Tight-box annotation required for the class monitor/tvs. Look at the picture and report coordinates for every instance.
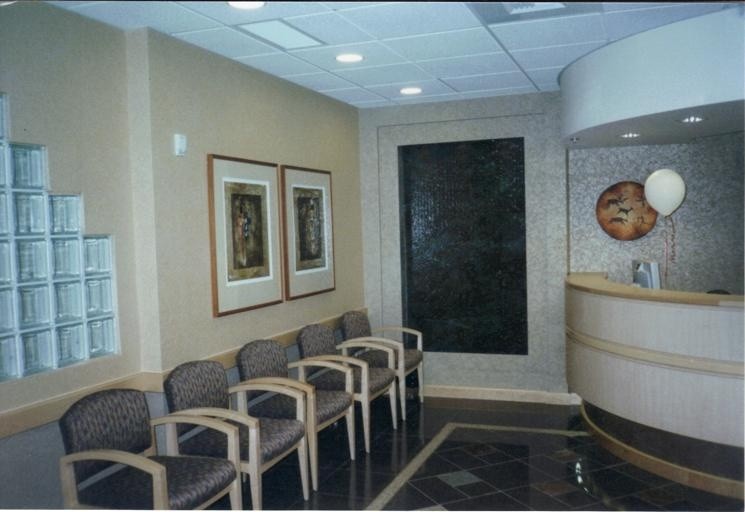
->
[632,259,661,290]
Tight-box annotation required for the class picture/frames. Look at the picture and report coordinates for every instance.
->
[208,154,287,318]
[281,164,336,301]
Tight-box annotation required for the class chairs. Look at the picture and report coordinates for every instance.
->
[164,360,310,510]
[59,389,245,510]
[297,324,399,453]
[234,340,357,492]
[340,311,424,421]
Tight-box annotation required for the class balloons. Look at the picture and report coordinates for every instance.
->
[643,169,686,218]
